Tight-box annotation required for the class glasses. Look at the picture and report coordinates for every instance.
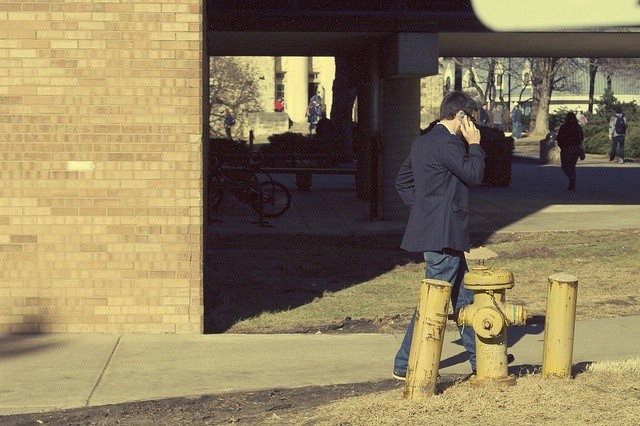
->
[463,109,476,124]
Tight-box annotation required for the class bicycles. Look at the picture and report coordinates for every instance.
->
[209,150,292,218]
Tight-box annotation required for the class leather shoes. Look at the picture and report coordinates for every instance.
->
[393,368,441,381]
[471,353,515,376]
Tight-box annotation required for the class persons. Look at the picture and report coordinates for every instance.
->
[556,112,584,190]
[480,104,489,127]
[223,109,235,139]
[392,91,514,381]
[273,97,284,112]
[510,101,522,138]
[304,91,326,134]
[578,111,588,125]
[574,110,581,120]
[609,106,626,164]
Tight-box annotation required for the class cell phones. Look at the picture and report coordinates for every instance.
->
[458,112,471,128]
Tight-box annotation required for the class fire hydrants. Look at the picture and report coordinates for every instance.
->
[448,258,533,386]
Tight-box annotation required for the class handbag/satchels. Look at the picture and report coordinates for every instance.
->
[579,147,585,160]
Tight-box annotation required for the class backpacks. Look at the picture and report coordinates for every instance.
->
[614,113,626,133]
